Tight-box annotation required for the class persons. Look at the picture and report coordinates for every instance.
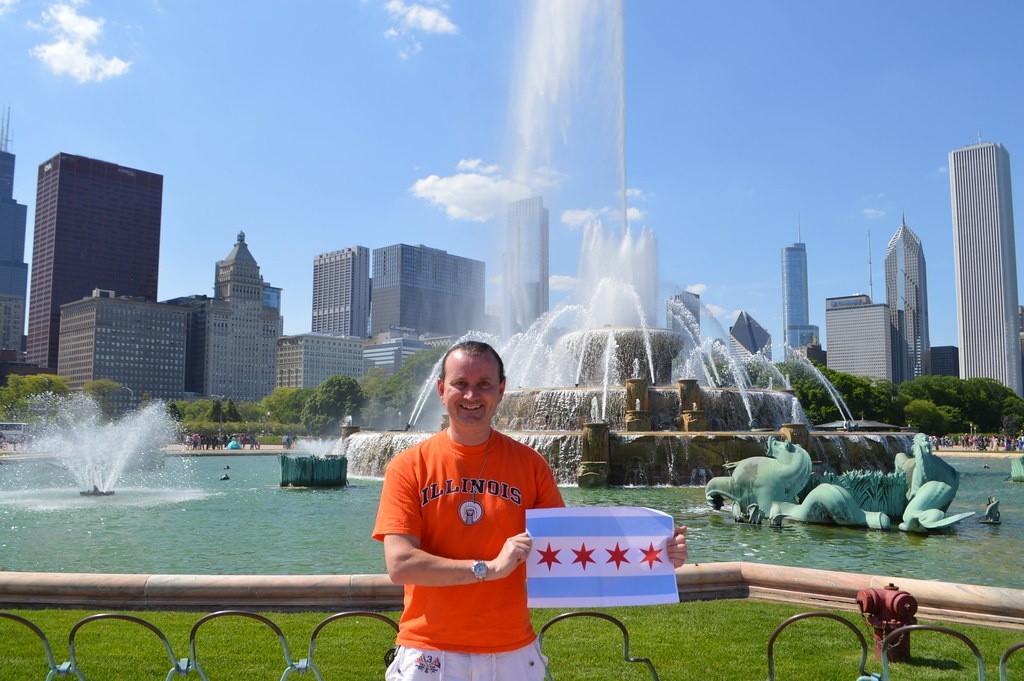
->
[176,431,332,450]
[373,341,688,681]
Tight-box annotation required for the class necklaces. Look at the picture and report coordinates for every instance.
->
[447,426,493,506]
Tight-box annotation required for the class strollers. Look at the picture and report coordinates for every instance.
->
[977,439,987,451]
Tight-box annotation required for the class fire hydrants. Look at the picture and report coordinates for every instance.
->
[855,582,919,664]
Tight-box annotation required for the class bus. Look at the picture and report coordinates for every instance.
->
[0,423,30,446]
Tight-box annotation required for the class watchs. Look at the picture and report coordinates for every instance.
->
[472,559,488,582]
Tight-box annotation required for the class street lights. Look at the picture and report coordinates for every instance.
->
[123,387,133,411]
[969,422,973,436]
[267,411,271,436]
[211,395,224,437]
[974,427,977,437]
